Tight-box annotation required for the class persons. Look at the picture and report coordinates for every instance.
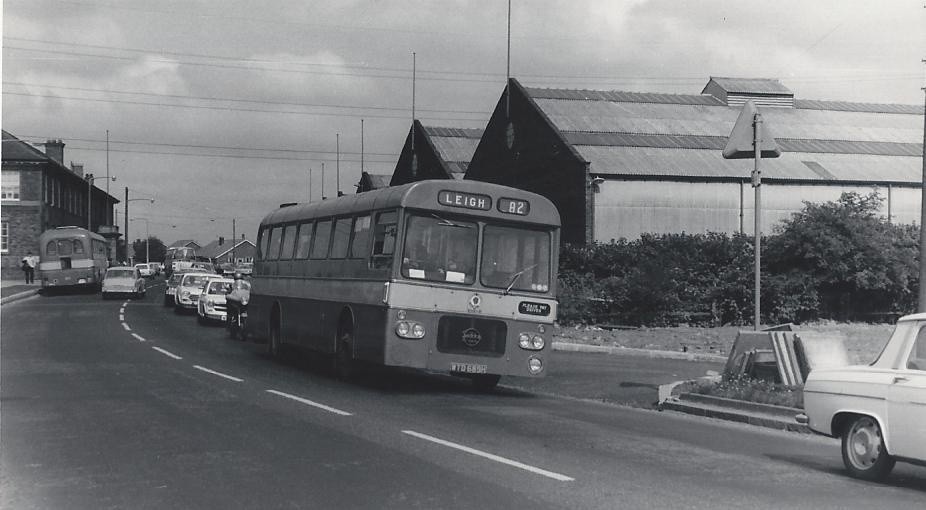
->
[224,273,245,329]
[19,250,37,284]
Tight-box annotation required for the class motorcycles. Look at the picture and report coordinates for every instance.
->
[226,305,249,341]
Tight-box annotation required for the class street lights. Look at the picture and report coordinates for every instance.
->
[86,175,116,232]
[208,218,236,259]
[114,198,155,230]
[128,218,150,268]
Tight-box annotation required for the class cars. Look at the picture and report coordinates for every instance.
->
[793,312,926,481]
[101,254,254,325]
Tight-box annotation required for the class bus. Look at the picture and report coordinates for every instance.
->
[38,225,110,290]
[240,179,563,389]
